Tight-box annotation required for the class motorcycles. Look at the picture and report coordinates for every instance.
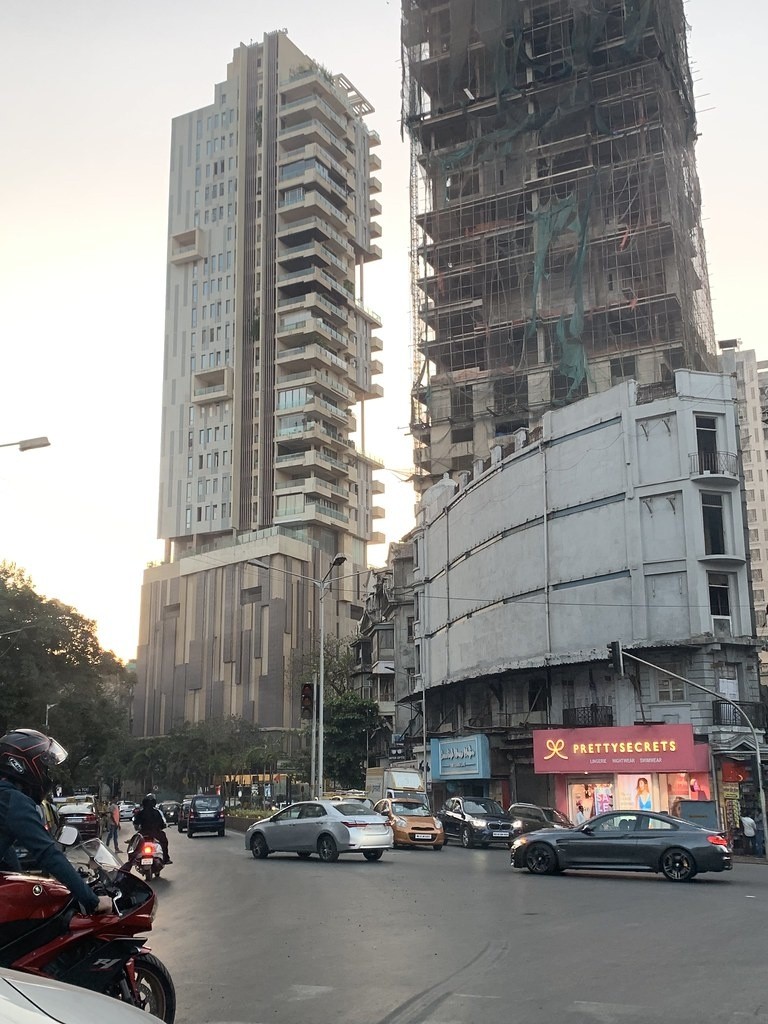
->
[0,827,178,1024]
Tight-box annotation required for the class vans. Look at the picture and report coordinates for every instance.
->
[187,793,226,838]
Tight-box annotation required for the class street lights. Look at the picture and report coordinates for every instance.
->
[384,666,427,790]
[246,551,395,803]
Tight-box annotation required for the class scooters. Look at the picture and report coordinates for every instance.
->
[128,817,171,881]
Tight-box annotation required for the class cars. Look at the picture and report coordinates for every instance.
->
[507,802,576,833]
[158,802,181,825]
[58,802,105,845]
[177,795,209,834]
[330,795,376,810]
[244,801,394,863]
[15,800,56,877]
[372,798,443,850]
[117,800,136,822]
[509,809,736,882]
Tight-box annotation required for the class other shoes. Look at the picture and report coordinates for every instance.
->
[164,860,172,864]
[115,849,123,853]
[755,855,763,859]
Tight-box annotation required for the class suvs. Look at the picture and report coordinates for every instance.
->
[435,795,522,850]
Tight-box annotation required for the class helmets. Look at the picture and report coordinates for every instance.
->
[142,794,156,807]
[0,728,69,806]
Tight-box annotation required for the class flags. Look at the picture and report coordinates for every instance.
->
[274,774,280,781]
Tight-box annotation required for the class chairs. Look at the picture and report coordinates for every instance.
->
[619,819,636,830]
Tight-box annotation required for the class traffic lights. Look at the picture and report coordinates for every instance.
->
[299,681,313,720]
[606,642,625,676]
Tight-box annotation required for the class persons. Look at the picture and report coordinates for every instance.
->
[635,778,653,829]
[0,728,112,916]
[740,807,768,858]
[619,819,630,829]
[133,792,173,864]
[106,795,123,853]
[577,806,585,824]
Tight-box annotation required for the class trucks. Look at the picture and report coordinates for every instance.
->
[366,767,431,813]
